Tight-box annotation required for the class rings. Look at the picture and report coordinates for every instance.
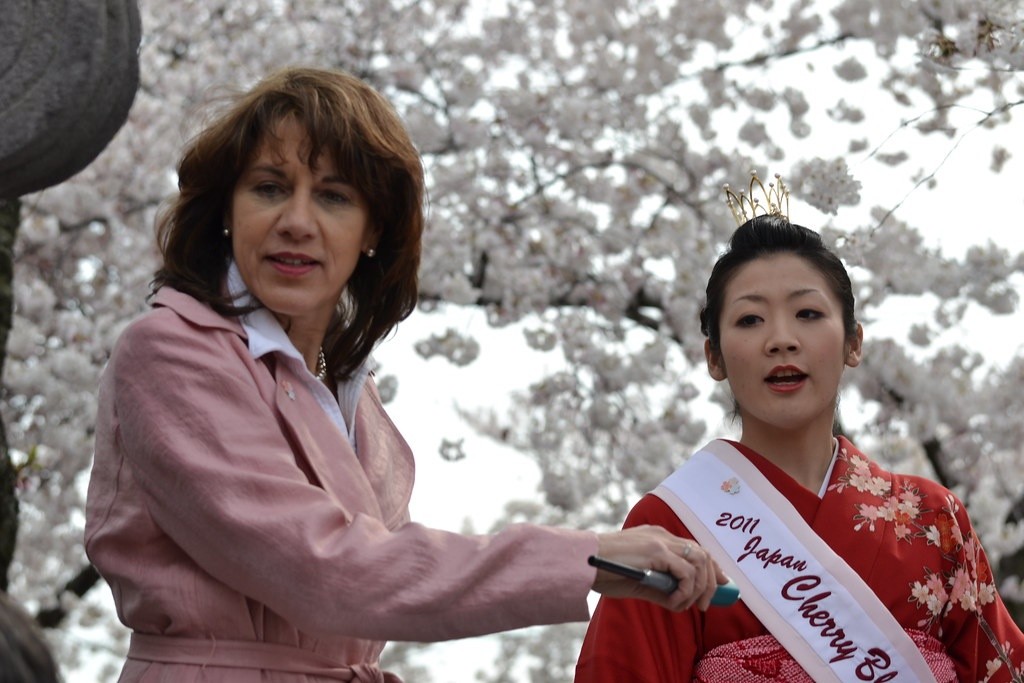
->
[683,544,692,559]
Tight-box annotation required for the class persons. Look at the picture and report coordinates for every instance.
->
[85,64,728,683]
[574,170,1024,683]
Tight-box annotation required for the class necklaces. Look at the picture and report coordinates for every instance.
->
[313,345,328,383]
